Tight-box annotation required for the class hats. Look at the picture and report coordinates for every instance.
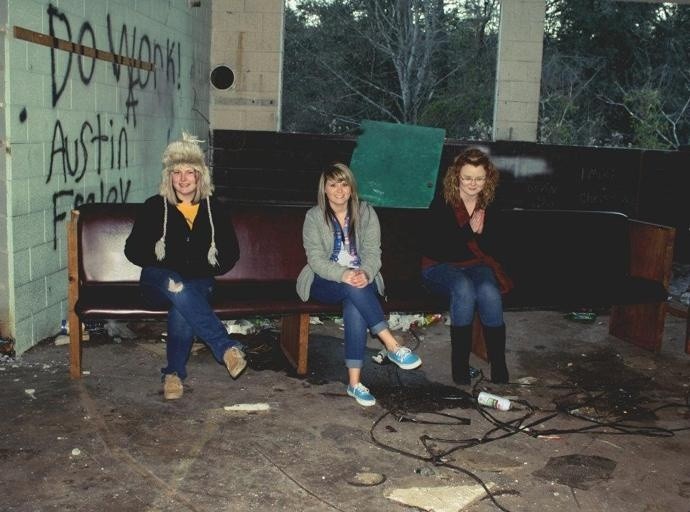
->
[160,129,214,201]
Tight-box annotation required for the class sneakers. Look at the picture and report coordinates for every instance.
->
[347,383,376,407]
[163,374,184,400]
[387,346,422,370]
[222,346,248,379]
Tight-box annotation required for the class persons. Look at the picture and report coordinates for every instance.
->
[418,149,513,385]
[124,140,248,401]
[295,162,423,407]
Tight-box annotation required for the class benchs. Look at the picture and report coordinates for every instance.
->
[67,203,677,378]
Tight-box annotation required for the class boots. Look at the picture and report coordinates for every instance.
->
[483,323,509,385]
[451,322,472,385]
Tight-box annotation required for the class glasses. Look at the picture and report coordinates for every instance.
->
[458,175,486,185]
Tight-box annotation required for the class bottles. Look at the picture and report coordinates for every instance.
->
[563,311,597,321]
[476,391,514,412]
